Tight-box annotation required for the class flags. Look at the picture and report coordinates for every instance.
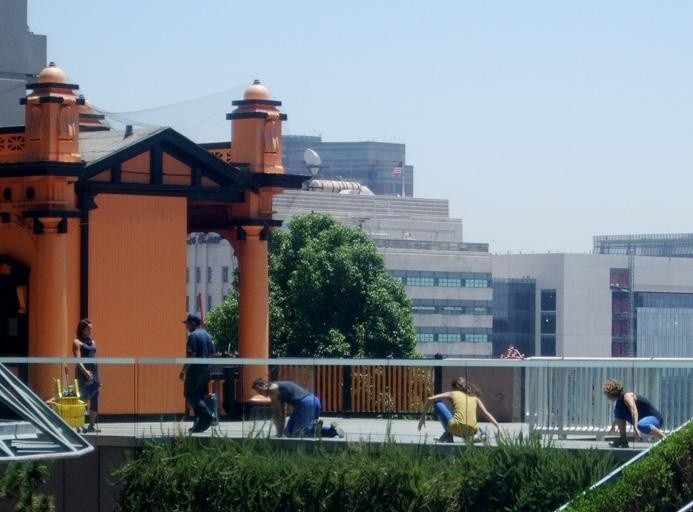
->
[392,158,402,176]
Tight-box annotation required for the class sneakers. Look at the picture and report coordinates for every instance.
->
[434,435,453,443]
[88,425,102,433]
[331,421,345,437]
[608,438,628,448]
[189,412,218,433]
[314,419,323,438]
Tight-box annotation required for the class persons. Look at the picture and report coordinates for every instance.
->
[601,377,665,449]
[178,312,218,433]
[71,318,102,433]
[249,375,346,439]
[416,375,504,444]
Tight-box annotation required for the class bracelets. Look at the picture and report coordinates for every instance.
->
[421,416,425,420]
[180,370,184,373]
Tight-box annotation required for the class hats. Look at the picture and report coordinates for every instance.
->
[182,314,202,326]
